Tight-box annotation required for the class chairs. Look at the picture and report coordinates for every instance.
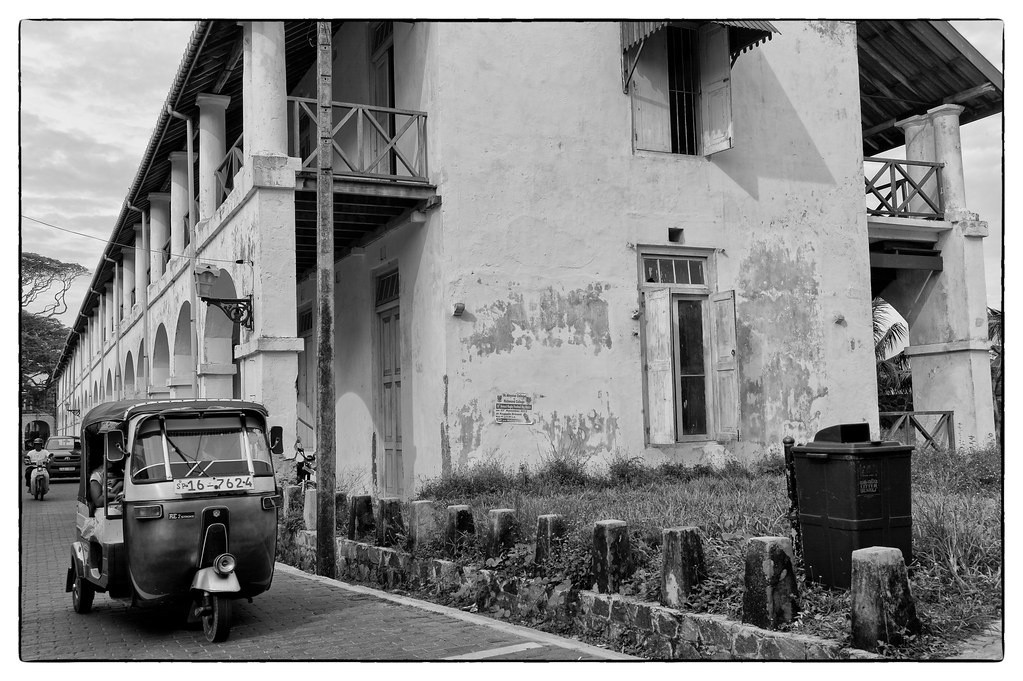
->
[49,441,60,449]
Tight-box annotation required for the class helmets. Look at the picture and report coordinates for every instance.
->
[33,438,44,447]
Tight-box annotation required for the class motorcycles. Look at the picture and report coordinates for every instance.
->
[24,452,54,501]
[66,399,285,644]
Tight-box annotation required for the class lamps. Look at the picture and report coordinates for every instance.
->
[452,302,465,316]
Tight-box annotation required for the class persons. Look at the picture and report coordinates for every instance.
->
[86,459,125,516]
[24,438,52,493]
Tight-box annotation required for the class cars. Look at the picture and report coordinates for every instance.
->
[45,435,82,478]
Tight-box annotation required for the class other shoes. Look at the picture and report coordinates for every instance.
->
[27,488,31,493]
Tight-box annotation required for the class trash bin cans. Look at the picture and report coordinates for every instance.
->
[790,423,917,593]
